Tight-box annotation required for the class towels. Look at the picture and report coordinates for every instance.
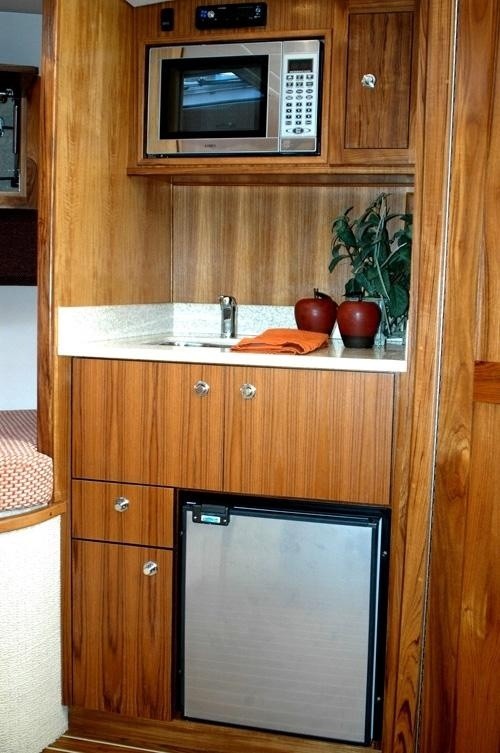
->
[231,328,330,354]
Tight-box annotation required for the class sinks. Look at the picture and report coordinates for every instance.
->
[141,337,242,349]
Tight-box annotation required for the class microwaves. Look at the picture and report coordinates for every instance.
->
[145,38,320,157]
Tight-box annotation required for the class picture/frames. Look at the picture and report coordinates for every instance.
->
[0,78,28,197]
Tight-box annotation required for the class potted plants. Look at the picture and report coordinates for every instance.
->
[329,190,407,349]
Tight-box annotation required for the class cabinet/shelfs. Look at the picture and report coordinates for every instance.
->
[70,477,176,723]
[38,0,498,374]
[70,357,395,509]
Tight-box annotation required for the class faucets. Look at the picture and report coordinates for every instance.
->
[217,295,238,338]
